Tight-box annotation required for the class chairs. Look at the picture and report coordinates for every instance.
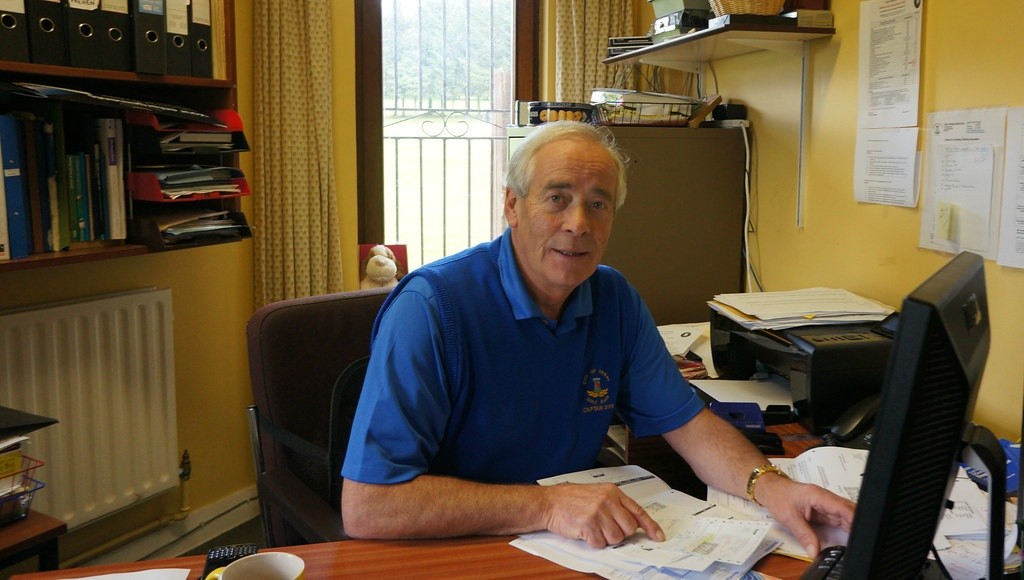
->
[247,290,395,548]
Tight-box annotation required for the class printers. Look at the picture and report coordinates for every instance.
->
[710,308,900,437]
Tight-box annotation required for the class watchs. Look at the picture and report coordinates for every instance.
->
[743,464,792,509]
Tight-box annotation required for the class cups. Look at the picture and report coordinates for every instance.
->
[206,550,306,580]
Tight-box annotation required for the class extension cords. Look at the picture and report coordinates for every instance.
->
[698,119,750,128]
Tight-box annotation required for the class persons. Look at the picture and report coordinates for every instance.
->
[338,119,861,563]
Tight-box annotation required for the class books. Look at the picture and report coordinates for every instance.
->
[0,113,136,261]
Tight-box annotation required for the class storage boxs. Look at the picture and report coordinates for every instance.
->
[646,0,785,46]
[0,475,46,529]
[588,101,693,127]
[0,455,45,499]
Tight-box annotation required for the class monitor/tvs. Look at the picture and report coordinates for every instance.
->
[841,251,990,580]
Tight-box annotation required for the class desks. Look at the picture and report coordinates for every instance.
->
[11,335,1024,580]
[0,503,68,580]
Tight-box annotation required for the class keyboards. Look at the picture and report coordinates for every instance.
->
[799,545,848,580]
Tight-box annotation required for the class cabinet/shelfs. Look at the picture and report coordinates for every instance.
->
[1,0,251,270]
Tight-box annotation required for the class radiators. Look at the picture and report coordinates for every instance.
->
[1,286,182,535]
